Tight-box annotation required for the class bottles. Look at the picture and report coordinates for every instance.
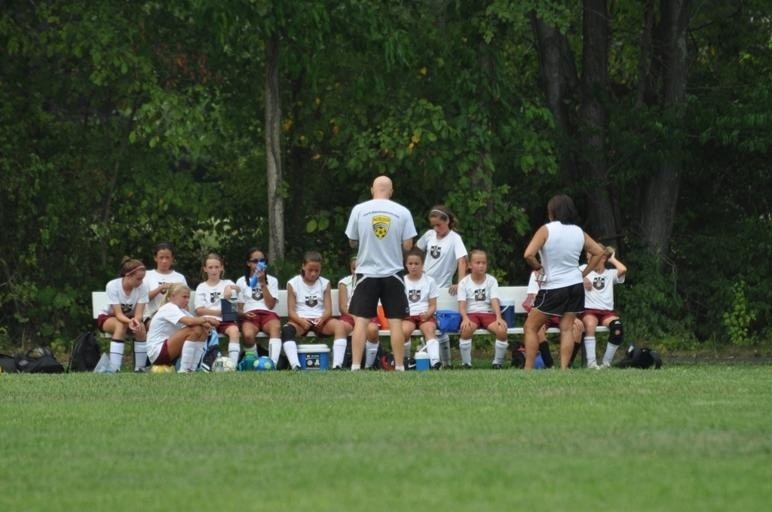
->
[250,260,267,288]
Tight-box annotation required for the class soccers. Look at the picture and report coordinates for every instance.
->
[252,356,276,371]
[211,355,236,374]
[151,364,177,375]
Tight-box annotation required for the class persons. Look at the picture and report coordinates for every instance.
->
[338,253,381,369]
[236,247,282,370]
[578,241,627,369]
[195,253,241,372]
[282,251,348,371]
[413,206,469,370]
[523,196,604,369]
[522,268,585,369]
[344,177,419,372]
[141,243,194,372]
[144,282,219,373]
[96,254,149,373]
[456,249,508,369]
[399,246,444,371]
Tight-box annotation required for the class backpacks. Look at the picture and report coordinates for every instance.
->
[617,345,662,369]
[67,330,101,373]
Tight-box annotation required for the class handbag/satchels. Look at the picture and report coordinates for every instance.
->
[15,341,65,373]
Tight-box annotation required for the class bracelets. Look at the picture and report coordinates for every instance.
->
[535,265,541,272]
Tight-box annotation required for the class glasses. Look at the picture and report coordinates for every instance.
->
[248,258,266,263]
